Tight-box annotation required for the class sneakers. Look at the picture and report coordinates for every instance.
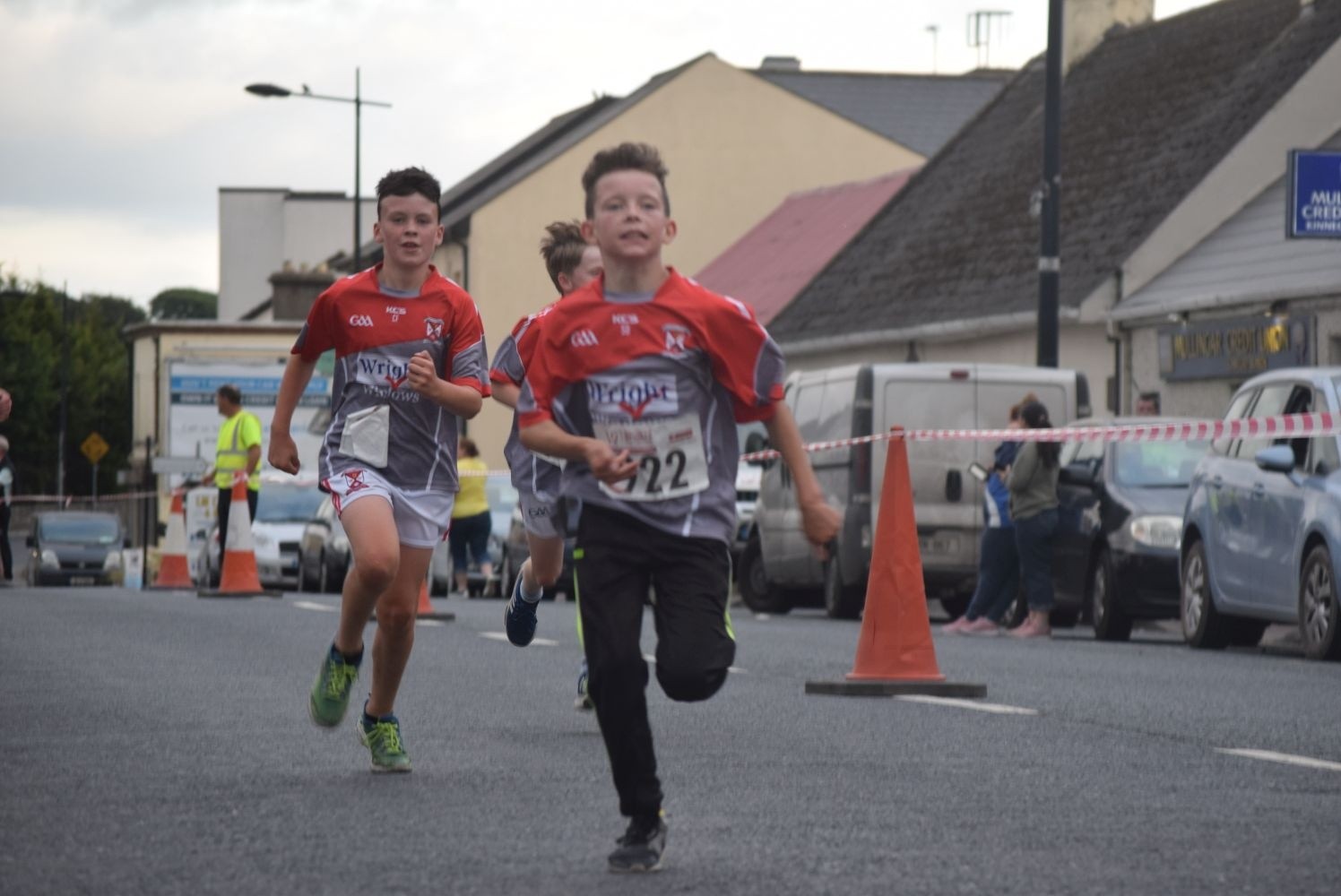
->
[309,636,364,726]
[357,694,412,773]
[607,812,669,872]
[573,666,594,709]
[504,566,540,647]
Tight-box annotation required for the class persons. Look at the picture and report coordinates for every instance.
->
[0,435,12,586]
[200,384,263,588]
[442,437,498,601]
[514,142,842,873]
[940,392,1063,638]
[269,166,492,773]
[489,218,604,711]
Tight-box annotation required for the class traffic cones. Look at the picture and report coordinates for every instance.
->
[198,469,282,600]
[803,425,990,699]
[149,496,198,591]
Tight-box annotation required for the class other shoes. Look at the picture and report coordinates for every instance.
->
[448,590,469,599]
[483,578,496,597]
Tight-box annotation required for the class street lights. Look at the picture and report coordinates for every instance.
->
[243,64,394,276]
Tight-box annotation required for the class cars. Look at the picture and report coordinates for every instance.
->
[1175,364,1341,660]
[23,510,130,587]
[1057,414,1220,642]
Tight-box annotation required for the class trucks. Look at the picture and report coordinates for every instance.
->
[245,360,1084,619]
